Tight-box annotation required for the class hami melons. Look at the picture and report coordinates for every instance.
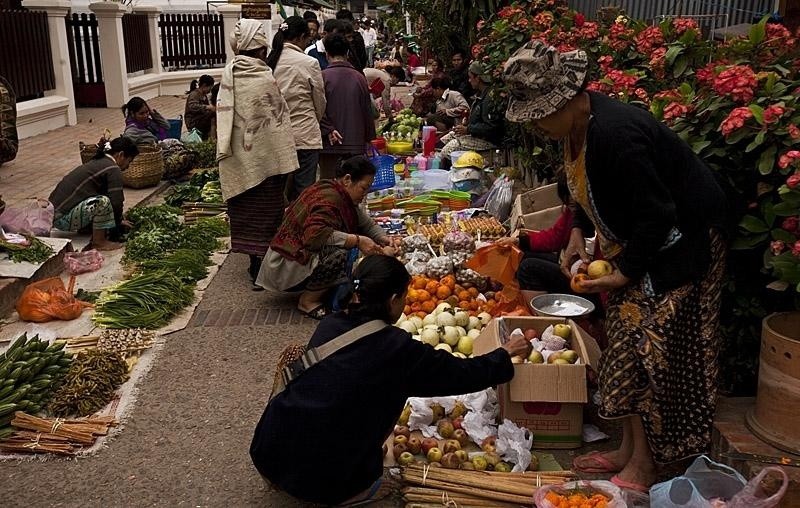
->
[392,302,491,359]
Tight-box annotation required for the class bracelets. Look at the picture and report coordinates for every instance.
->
[355,234,360,247]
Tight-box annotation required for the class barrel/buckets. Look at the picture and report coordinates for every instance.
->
[168,118,182,141]
[371,139,386,155]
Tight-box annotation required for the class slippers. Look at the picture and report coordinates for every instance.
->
[573,450,622,473]
[610,472,661,493]
[298,303,333,320]
[342,478,385,506]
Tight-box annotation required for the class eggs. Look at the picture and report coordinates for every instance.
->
[412,216,507,251]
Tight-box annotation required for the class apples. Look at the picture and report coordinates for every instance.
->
[508,324,577,364]
[587,259,612,280]
[393,399,540,472]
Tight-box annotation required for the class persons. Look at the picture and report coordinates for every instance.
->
[0,74,19,218]
[185,75,222,141]
[121,97,181,145]
[410,52,504,146]
[47,136,139,250]
[502,39,727,504]
[267,9,379,189]
[514,168,609,323]
[254,155,399,319]
[215,19,300,290]
[249,254,533,508]
[363,44,421,121]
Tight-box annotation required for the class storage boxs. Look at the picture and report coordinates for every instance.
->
[471,315,605,449]
[507,178,566,234]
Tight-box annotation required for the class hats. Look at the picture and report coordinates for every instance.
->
[467,60,492,82]
[501,38,589,123]
[451,150,484,195]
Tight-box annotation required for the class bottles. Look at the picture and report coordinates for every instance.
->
[383,131,413,143]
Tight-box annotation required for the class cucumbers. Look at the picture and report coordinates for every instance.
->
[0,332,76,439]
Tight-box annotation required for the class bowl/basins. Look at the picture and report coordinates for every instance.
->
[387,142,412,153]
[368,189,471,224]
[529,292,596,321]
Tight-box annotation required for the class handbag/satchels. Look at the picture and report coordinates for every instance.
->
[268,343,307,402]
[181,126,203,143]
[1,196,55,235]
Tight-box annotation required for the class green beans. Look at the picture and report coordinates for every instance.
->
[48,346,130,417]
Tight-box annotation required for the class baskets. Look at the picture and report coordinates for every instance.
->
[79,127,112,164]
[122,143,164,189]
[366,145,395,192]
[166,114,182,140]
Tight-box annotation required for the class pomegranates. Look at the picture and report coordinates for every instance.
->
[577,262,588,273]
[570,273,589,294]
